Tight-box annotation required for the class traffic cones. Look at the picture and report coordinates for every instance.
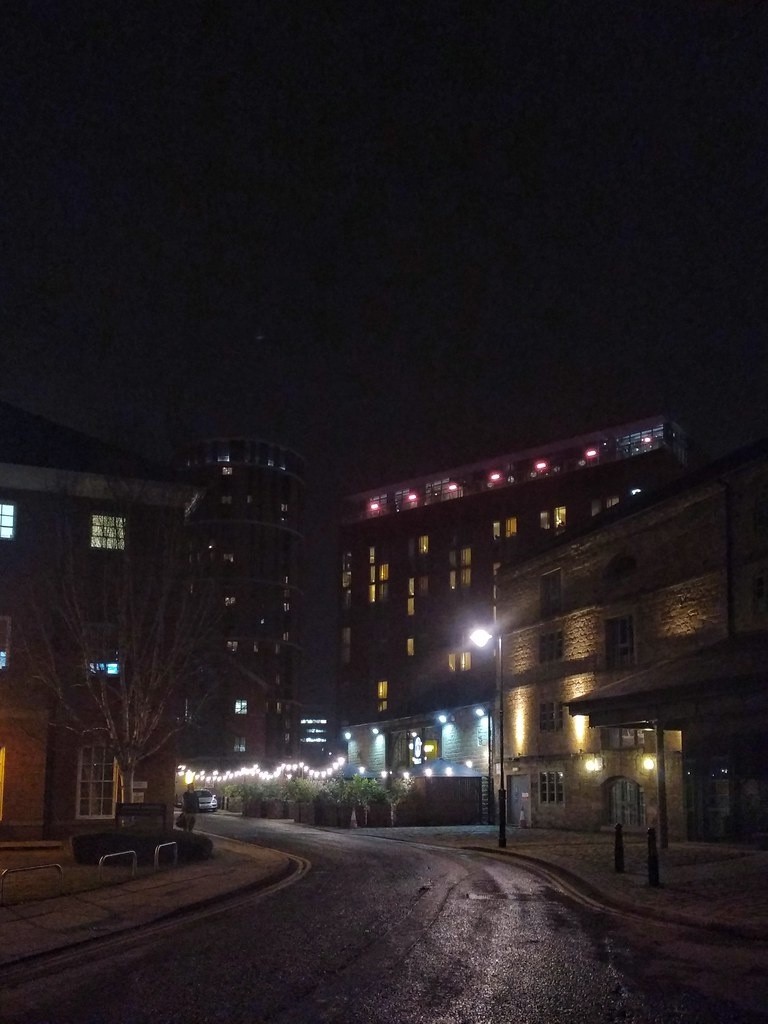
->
[349,806,358,829]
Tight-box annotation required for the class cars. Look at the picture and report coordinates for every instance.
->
[194,789,218,812]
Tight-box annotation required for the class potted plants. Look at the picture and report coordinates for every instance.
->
[225,777,415,827]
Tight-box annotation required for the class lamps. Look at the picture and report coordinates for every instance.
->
[517,752,523,756]
[579,748,586,753]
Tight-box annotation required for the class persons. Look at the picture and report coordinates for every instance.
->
[183,783,199,833]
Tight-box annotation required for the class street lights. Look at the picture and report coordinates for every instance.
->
[468,628,508,847]
